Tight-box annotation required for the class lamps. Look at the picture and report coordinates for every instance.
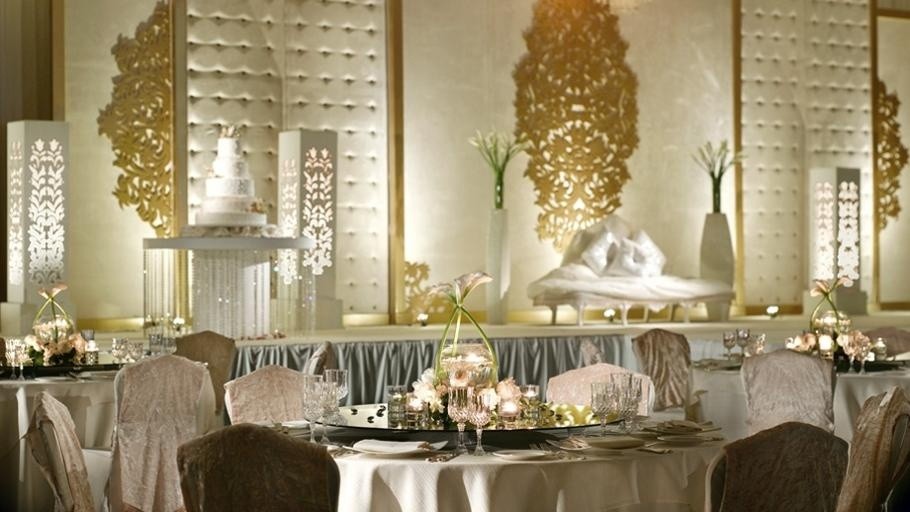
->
[809,165,861,294]
[279,125,344,336]
[7,119,70,307]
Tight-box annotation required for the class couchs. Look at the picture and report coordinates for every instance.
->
[527,215,738,324]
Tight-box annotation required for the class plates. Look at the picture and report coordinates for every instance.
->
[346,435,451,460]
[282,420,311,429]
[714,360,742,368]
[491,417,713,463]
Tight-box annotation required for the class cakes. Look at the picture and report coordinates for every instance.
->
[193,137,267,226]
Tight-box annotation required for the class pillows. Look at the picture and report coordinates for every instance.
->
[609,238,657,278]
[637,231,667,263]
[582,230,621,274]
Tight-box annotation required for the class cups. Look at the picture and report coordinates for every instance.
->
[147,325,163,354]
[747,333,766,355]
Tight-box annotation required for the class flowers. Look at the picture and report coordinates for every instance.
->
[806,272,850,328]
[31,277,71,327]
[468,127,523,209]
[687,136,750,210]
[422,268,497,378]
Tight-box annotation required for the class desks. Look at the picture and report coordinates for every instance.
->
[137,236,310,337]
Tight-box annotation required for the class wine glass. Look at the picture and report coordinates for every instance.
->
[5,333,27,382]
[443,385,495,463]
[300,367,350,449]
[589,369,646,435]
[112,336,143,364]
[721,328,750,364]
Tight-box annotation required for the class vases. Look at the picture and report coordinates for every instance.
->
[697,210,739,321]
[482,208,513,323]
